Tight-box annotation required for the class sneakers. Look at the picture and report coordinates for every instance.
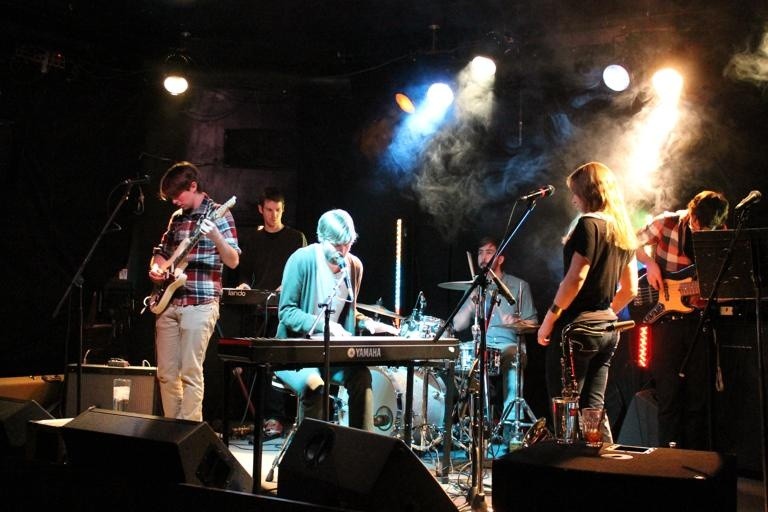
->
[248,417,284,445]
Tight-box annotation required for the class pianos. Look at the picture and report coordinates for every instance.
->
[223,286,281,307]
[217,335,460,364]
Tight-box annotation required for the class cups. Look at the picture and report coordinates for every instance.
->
[111,378,131,412]
[583,407,606,447]
[551,395,579,445]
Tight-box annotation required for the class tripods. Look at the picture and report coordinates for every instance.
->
[435,355,494,477]
[491,331,539,441]
[390,365,468,458]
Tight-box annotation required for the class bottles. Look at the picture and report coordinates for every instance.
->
[473,493,488,511]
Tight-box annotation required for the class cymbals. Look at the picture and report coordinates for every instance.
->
[357,303,404,319]
[439,282,499,291]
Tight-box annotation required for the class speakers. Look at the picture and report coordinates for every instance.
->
[64,363,165,418]
[279,415,459,512]
[60,405,255,494]
[0,397,57,454]
[492,438,738,512]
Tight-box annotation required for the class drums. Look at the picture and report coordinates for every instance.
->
[399,316,455,340]
[337,366,445,442]
[455,343,500,377]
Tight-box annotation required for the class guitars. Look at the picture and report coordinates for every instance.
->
[149,195,236,314]
[629,273,700,325]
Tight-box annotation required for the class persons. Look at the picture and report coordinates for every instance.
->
[272,209,374,434]
[537,161,640,444]
[148,161,243,423]
[603,191,729,450]
[452,237,539,439]
[235,187,308,434]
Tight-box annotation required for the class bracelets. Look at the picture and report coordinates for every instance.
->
[643,256,654,265]
[468,303,474,312]
[549,303,563,316]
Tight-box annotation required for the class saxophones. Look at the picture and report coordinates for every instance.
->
[523,319,635,449]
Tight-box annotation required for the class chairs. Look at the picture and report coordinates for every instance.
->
[258,318,345,485]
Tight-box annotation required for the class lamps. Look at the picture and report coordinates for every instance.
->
[145,28,195,97]
[599,41,691,99]
[391,36,509,118]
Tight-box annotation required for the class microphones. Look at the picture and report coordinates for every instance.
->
[734,191,761,210]
[125,175,153,184]
[489,267,517,306]
[337,257,355,301]
[517,185,556,202]
